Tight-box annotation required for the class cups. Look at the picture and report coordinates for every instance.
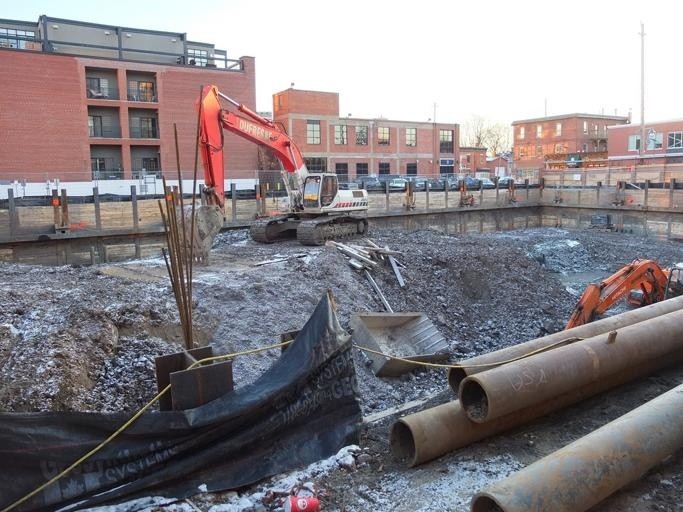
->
[284,495,320,512]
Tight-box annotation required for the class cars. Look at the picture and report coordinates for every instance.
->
[338,172,516,193]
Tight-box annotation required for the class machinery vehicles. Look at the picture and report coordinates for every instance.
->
[565,257,683,332]
[170,81,372,266]
